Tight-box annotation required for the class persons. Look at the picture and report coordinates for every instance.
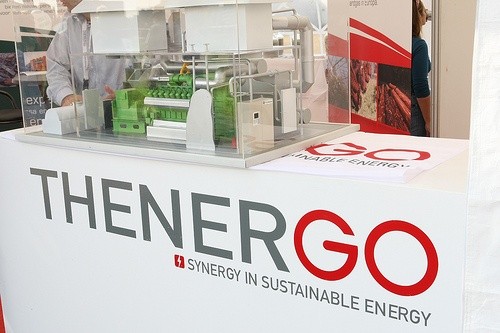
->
[0,53,25,84]
[412,0,434,138]
[45,0,129,108]
